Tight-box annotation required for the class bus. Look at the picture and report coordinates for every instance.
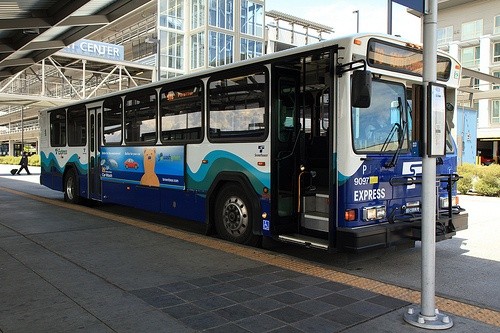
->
[39,33,467,264]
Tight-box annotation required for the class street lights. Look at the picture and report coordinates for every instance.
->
[62,75,72,100]
[144,38,160,81]
[353,10,359,33]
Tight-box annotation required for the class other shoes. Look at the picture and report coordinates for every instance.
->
[17,173,21,175]
[28,173,32,175]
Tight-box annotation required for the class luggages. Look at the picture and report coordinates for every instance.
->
[10,164,23,175]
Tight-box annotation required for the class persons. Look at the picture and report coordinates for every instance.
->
[27,152,31,156]
[364,104,395,143]
[15,152,31,176]
